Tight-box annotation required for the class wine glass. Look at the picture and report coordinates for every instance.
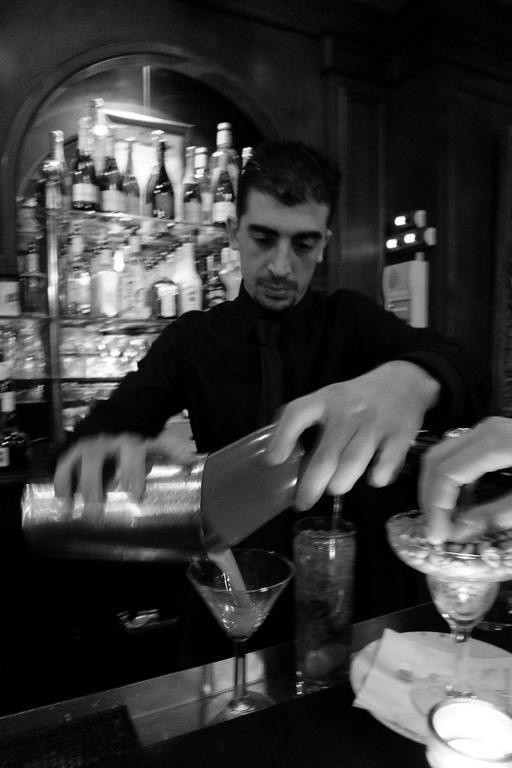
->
[387,505,512,720]
[185,545,299,729]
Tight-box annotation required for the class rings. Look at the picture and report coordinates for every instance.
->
[429,472,459,510]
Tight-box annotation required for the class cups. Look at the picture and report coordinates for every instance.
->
[199,421,313,546]
[21,449,211,562]
[290,513,358,697]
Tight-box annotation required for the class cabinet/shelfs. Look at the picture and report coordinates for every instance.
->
[0,157,253,450]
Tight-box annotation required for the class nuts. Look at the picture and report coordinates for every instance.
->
[388,513,512,580]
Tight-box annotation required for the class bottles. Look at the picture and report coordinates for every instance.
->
[0,95,253,467]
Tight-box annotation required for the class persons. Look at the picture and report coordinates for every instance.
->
[35,135,460,662]
[412,412,512,549]
